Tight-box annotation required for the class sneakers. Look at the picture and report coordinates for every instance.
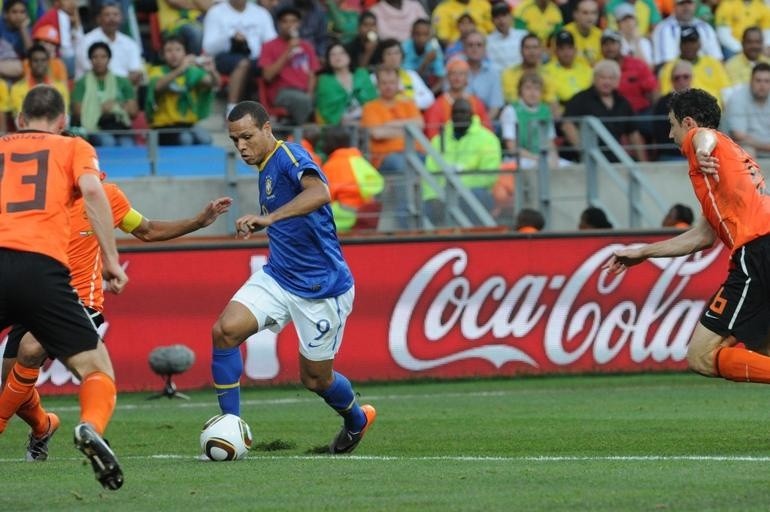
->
[72,423,125,493]
[25,412,61,463]
[327,403,378,454]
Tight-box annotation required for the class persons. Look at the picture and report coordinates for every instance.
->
[603,89,770,385]
[202,101,377,459]
[0,83,131,491]
[0,129,233,461]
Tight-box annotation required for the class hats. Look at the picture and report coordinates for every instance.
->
[32,24,59,43]
[599,29,622,44]
[613,3,636,22]
[553,31,575,48]
[680,25,700,42]
[490,2,511,18]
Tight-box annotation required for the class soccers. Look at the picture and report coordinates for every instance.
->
[200,413,251,461]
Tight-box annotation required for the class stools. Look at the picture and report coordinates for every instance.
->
[145,344,195,401]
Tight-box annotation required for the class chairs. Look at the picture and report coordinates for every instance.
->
[198,47,229,96]
[149,12,167,64]
[131,111,150,145]
[256,77,290,123]
[313,107,327,126]
